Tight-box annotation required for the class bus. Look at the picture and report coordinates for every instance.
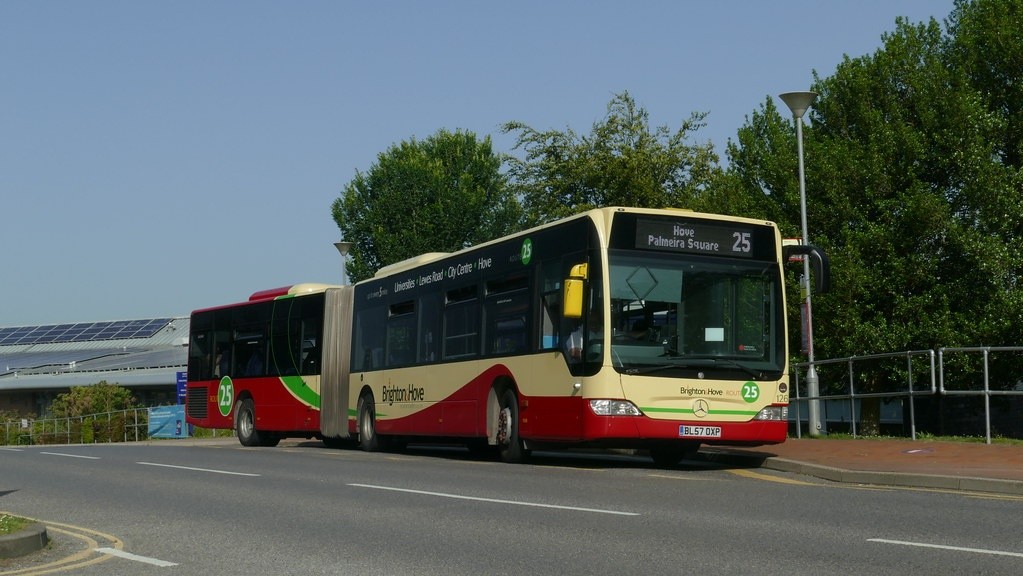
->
[186,204,833,464]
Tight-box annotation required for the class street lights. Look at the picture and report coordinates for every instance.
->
[777,90,827,440]
[332,241,356,285]
